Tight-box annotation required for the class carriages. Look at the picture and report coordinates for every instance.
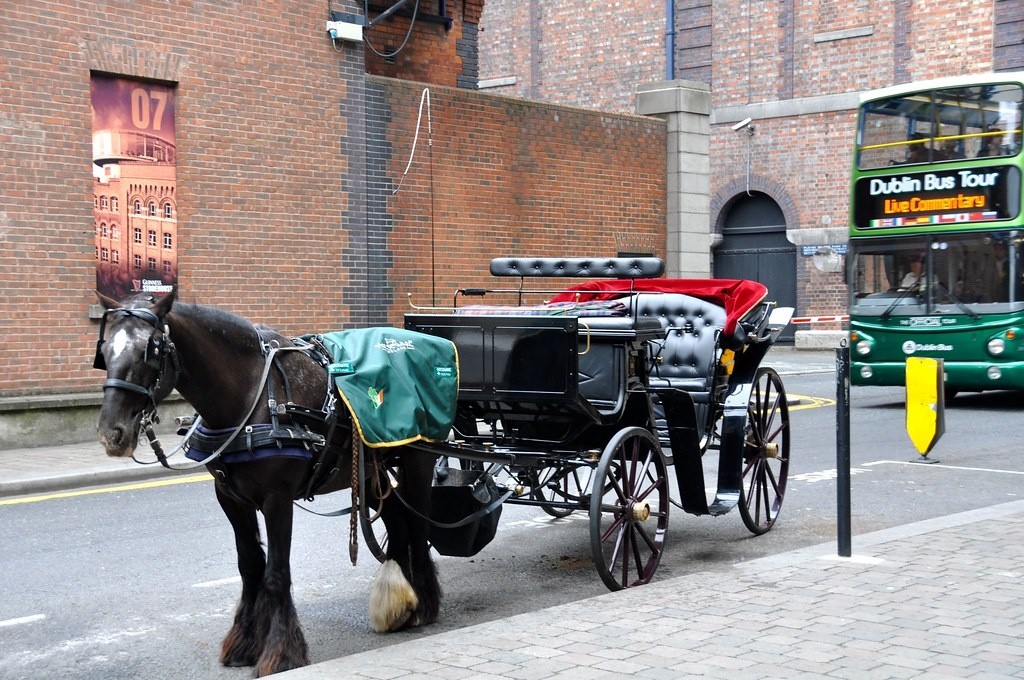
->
[93,258,796,680]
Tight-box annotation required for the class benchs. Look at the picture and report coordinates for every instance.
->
[454,256,664,408]
[610,293,728,438]
[888,143,1021,165]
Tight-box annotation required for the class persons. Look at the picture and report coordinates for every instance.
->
[886,131,1024,309]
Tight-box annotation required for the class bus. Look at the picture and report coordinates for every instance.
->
[841,71,1024,399]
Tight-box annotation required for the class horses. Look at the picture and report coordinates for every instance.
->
[94,286,460,678]
